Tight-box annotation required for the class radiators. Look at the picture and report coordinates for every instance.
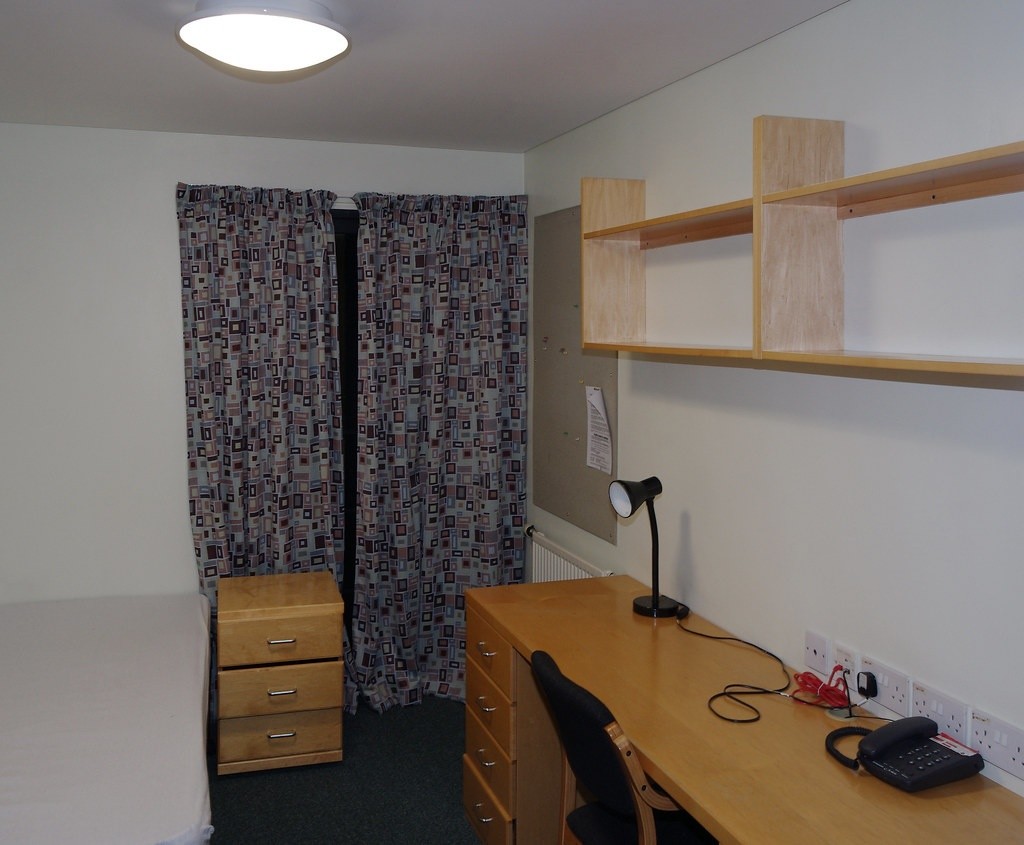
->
[521,523,613,582]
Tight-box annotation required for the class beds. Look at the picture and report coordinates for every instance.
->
[1,593,219,843]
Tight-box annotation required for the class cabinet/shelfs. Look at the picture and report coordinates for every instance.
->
[581,119,1023,388]
[217,571,343,777]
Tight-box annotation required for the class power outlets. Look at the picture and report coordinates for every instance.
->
[912,679,971,747]
[834,648,857,690]
[861,655,912,717]
[970,707,1024,782]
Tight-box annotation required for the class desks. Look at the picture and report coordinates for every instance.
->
[462,577,1023,845]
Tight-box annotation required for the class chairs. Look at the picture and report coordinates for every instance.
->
[530,649,721,845]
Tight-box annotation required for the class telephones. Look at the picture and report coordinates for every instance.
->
[856,715,985,794]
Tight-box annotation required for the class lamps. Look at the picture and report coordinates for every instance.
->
[609,475,679,618]
[178,1,351,73]
